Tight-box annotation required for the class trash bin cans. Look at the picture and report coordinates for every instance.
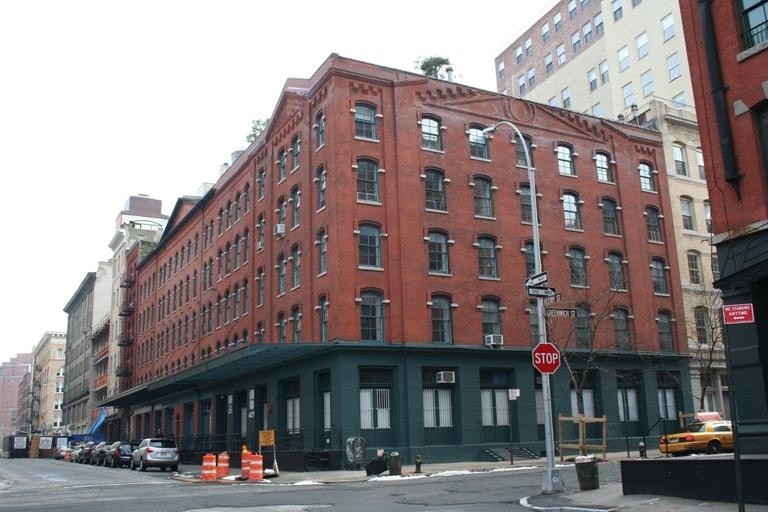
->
[574,455,600,490]
[389,456,401,475]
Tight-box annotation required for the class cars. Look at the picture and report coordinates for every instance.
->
[658,420,735,456]
[71,440,139,468]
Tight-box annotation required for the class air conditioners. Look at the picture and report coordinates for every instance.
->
[485,334,504,346]
[435,370,456,384]
[273,223,286,236]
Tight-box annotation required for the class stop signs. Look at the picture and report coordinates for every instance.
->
[531,343,560,375]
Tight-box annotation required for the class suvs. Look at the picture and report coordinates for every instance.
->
[130,437,180,472]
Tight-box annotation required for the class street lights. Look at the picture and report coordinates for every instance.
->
[482,119,566,493]
